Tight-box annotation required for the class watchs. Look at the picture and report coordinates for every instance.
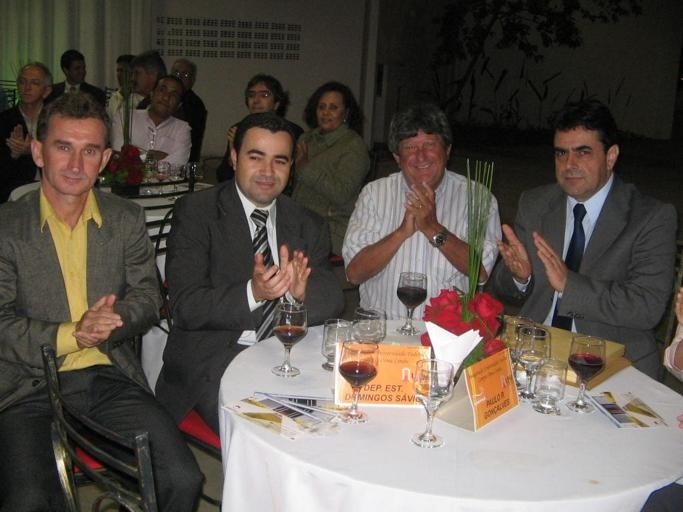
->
[428,226,449,249]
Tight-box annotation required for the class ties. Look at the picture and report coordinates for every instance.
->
[249,209,282,340]
[550,204,587,332]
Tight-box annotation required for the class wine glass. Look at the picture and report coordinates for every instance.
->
[338,338,379,424]
[411,360,454,448]
[515,327,551,399]
[397,272,427,336]
[504,316,535,388]
[568,336,604,412]
[352,309,387,344]
[273,303,309,376]
[141,158,204,202]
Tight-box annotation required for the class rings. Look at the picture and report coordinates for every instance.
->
[417,205,424,209]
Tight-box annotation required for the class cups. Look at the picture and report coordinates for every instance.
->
[534,359,569,413]
[322,318,350,372]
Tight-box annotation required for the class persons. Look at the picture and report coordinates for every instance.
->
[628,288,683,511]
[0,50,208,204]
[483,98,678,384]
[0,94,206,512]
[283,80,370,262]
[154,114,346,439]
[215,73,305,200]
[340,102,502,323]
